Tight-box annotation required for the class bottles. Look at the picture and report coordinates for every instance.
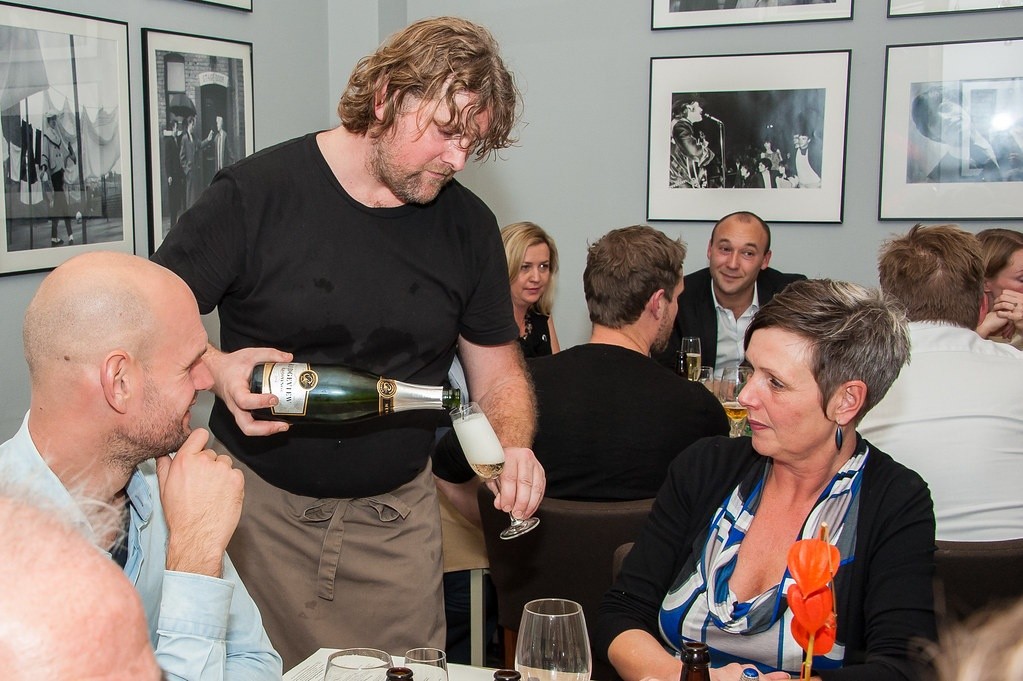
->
[494,671,521,681]
[738,668,759,681]
[251,362,461,428]
[386,667,415,681]
[680,642,711,681]
[673,351,688,381]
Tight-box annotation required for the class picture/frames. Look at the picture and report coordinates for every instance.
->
[886,1,1023,19]
[645,48,852,226]
[650,0,854,32]
[141,27,254,259]
[878,37,1023,221]
[0,2,136,279]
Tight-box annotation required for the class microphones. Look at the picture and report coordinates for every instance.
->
[704,114,722,124]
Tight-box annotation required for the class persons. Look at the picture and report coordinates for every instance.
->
[151,16,546,675]
[856,219,1023,542]
[0,248,285,681]
[0,452,164,681]
[912,85,1023,181]
[430,221,562,669]
[670,94,822,188]
[39,112,77,245]
[162,115,234,228]
[591,279,940,681]
[653,212,808,400]
[972,227,1023,349]
[432,225,732,504]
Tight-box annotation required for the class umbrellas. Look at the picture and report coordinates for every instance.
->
[168,93,196,119]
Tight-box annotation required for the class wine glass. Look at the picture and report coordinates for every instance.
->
[719,366,754,438]
[448,402,540,541]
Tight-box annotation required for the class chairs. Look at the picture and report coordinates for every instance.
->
[936,533,1023,630]
[474,482,658,679]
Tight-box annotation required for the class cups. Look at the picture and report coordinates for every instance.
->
[514,598,592,681]
[698,366,714,395]
[323,648,393,681]
[679,337,701,382]
[404,648,449,681]
